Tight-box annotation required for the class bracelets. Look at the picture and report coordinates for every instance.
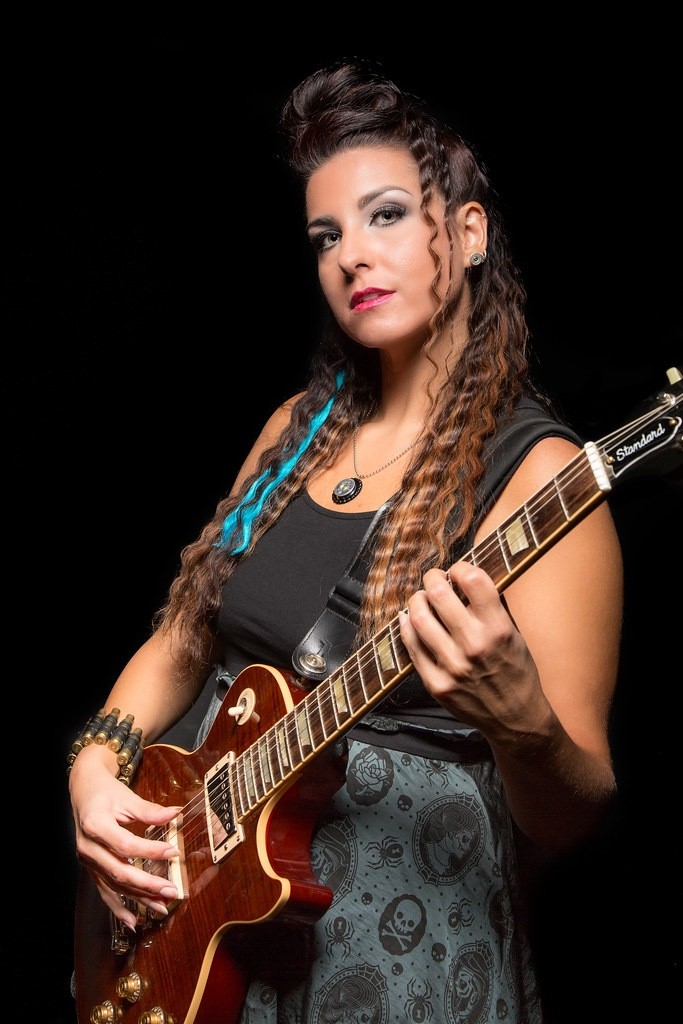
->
[65,707,147,786]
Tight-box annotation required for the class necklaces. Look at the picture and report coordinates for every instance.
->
[332,423,430,504]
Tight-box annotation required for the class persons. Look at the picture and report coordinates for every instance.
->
[65,59,623,1024]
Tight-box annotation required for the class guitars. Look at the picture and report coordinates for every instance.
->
[74,370,681,1024]
